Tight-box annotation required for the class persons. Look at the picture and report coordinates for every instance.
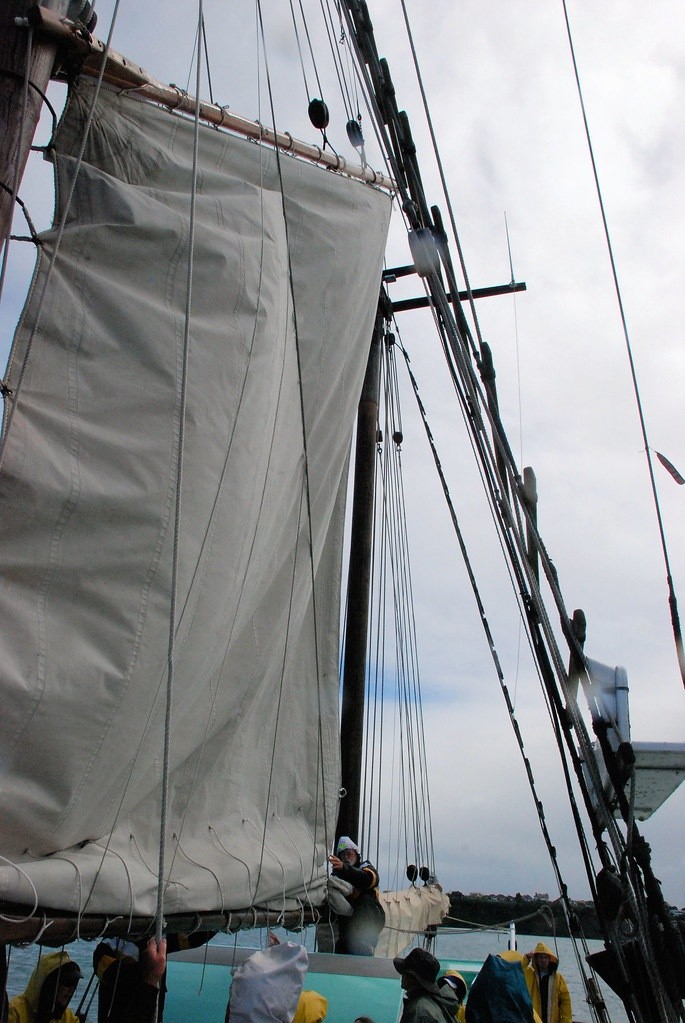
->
[441,969,467,1023]
[354,1017,373,1023]
[8,951,80,1023]
[316,836,386,957]
[292,990,328,1023]
[464,942,572,1023]
[224,941,309,1023]
[393,948,459,1023]
[99,935,167,1023]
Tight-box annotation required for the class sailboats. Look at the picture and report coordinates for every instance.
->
[1,0,685,1023]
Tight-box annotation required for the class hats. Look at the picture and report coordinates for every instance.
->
[393,948,440,993]
[337,836,359,855]
